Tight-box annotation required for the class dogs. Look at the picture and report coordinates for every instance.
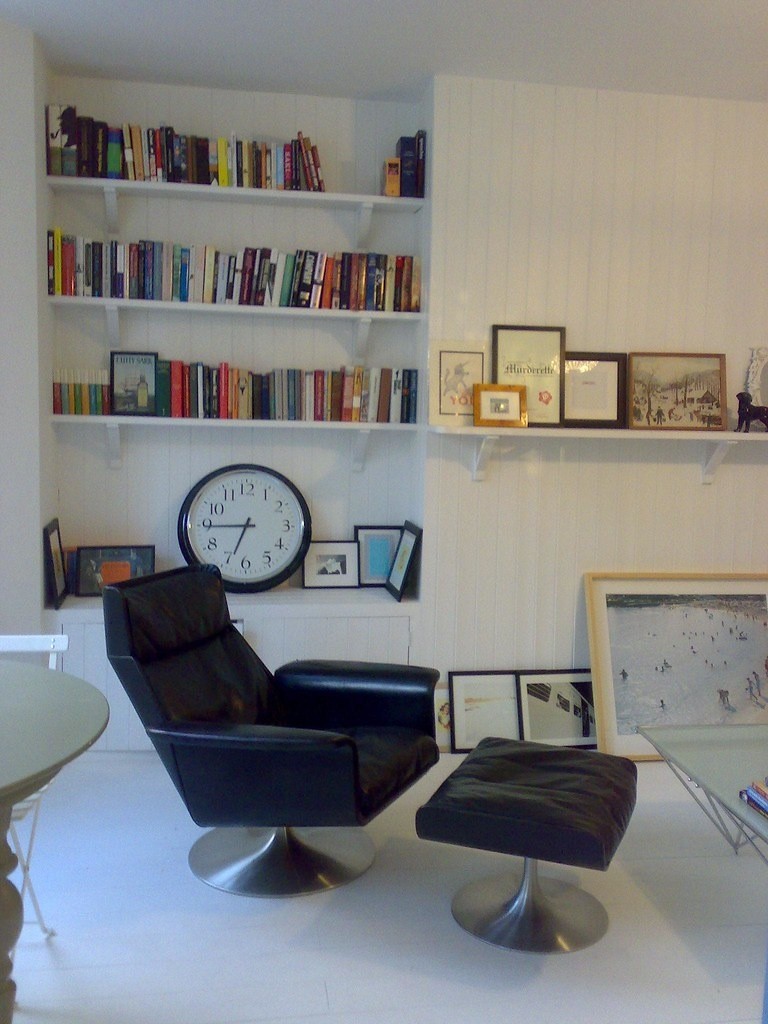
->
[733,392,768,432]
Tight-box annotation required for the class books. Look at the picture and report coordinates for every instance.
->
[47,226,421,312]
[53,350,418,423]
[385,129,426,198]
[47,102,326,192]
[739,777,768,819]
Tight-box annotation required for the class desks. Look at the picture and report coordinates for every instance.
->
[0,658,110,1024]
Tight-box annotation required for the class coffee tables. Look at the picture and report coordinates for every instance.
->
[637,725,768,866]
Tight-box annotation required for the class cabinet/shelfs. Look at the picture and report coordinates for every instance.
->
[41,177,421,469]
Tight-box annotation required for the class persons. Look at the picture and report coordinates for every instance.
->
[620,608,768,710]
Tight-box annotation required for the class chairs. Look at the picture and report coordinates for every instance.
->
[103,564,440,898]
[0,635,70,960]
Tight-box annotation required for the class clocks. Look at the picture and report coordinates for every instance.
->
[177,465,313,593]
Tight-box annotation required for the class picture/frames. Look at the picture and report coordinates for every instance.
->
[746,346,768,431]
[449,670,520,754]
[491,324,566,428]
[566,351,628,430]
[303,540,360,588]
[44,517,68,609]
[429,340,489,428]
[628,352,728,430]
[516,668,598,750]
[110,351,158,416]
[354,525,404,587]
[387,521,422,603]
[473,384,528,428]
[431,682,451,753]
[76,545,156,597]
[585,572,768,760]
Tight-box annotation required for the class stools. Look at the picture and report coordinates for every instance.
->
[416,737,638,948]
